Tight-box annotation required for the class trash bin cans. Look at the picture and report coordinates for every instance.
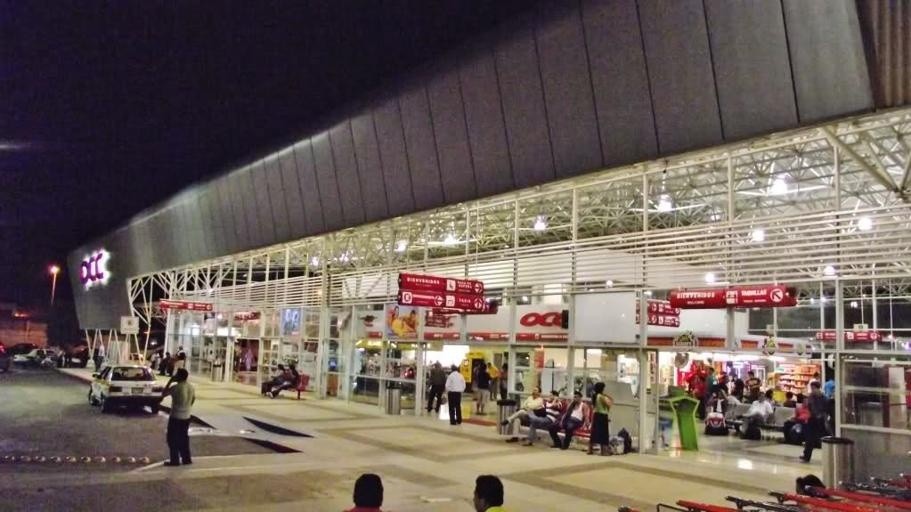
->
[821,436,855,490]
[212,364,222,382]
[385,387,401,415]
[496,399,518,435]
[155,357,162,370]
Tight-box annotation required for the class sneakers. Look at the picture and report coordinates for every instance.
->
[500,419,508,425]
[507,437,517,442]
[164,457,192,468]
[521,435,612,456]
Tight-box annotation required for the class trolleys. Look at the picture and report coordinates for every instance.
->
[618,471,911,512]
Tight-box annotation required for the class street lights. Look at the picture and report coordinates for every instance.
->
[48,265,61,305]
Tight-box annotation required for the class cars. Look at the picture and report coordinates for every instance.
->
[88,364,164,415]
[1,341,99,372]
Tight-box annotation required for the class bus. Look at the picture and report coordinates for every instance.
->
[140,330,165,359]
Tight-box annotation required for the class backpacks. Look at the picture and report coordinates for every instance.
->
[618,429,632,455]
[608,436,624,456]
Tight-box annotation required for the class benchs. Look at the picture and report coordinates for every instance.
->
[765,406,796,441]
[273,373,309,400]
[522,399,599,451]
[725,403,774,441]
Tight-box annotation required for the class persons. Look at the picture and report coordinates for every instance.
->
[472,361,508,416]
[163,352,175,376]
[403,310,415,333]
[472,474,510,512]
[342,473,383,512]
[176,346,185,369]
[207,350,221,366]
[262,364,298,399]
[93,341,104,371]
[445,365,467,425]
[161,367,196,466]
[697,367,834,463]
[390,305,403,336]
[243,348,254,370]
[500,381,613,456]
[794,475,828,504]
[150,349,160,369]
[427,362,446,413]
